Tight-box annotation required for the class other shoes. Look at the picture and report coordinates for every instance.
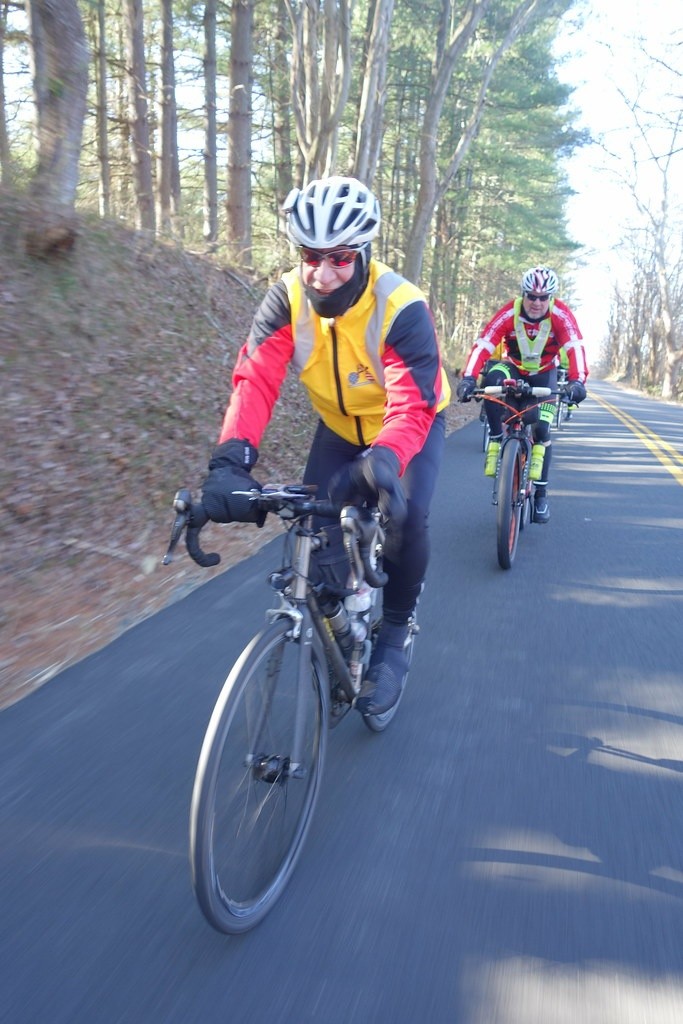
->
[355,623,413,717]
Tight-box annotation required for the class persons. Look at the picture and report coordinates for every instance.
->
[202,175,452,713]
[459,262,590,524]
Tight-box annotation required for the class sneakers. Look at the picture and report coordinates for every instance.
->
[534,490,550,523]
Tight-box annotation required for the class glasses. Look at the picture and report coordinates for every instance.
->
[301,243,369,268]
[525,292,550,302]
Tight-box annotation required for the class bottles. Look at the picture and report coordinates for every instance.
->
[484,439,500,476]
[322,566,375,658]
[528,440,546,481]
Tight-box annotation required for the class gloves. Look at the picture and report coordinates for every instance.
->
[566,380,587,402]
[328,445,408,536]
[201,437,268,528]
[456,376,478,402]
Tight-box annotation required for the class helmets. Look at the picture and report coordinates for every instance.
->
[282,176,381,249]
[521,267,556,293]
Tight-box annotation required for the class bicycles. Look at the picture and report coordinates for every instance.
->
[457,380,580,570]
[163,480,421,938]
[556,369,570,430]
